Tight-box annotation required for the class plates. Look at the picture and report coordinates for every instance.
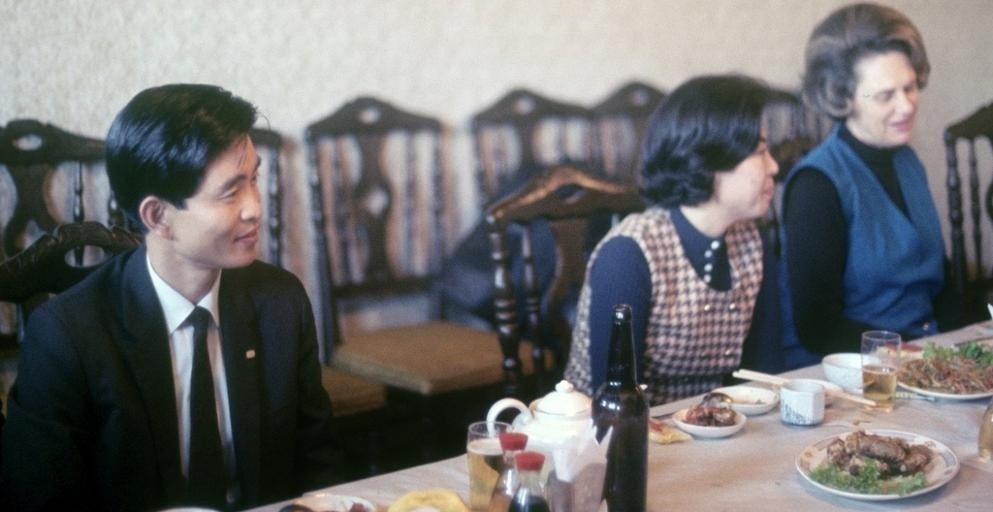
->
[897,348,993,400]
[672,407,748,438]
[798,428,963,499]
[289,495,378,511]
[712,385,779,416]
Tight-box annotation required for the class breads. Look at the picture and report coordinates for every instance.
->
[388,488,469,512]
[877,342,924,360]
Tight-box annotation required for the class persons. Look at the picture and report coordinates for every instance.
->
[560,72,820,416]
[776,2,960,360]
[1,83,343,512]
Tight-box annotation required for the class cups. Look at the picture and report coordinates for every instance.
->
[975,402,992,461]
[858,329,903,413]
[778,378,824,427]
[466,420,513,512]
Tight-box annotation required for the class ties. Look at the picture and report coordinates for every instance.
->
[188,306,227,511]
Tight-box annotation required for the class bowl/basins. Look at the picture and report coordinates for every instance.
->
[821,351,877,396]
[797,379,843,408]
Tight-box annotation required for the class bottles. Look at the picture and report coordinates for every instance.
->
[493,431,548,512]
[595,304,648,512]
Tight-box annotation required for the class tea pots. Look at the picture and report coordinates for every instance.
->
[486,381,606,512]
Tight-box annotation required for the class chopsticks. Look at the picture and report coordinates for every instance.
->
[730,368,878,408]
